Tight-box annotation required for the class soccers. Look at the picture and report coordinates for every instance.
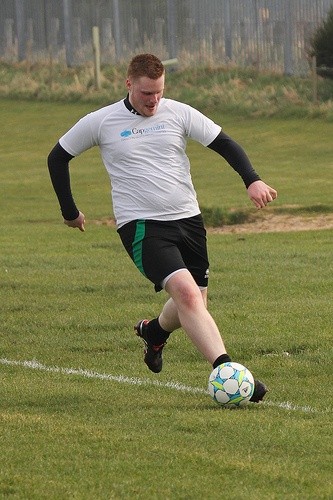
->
[208,361,255,408]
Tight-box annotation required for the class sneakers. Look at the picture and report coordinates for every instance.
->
[250,378,268,405]
[134,320,166,373]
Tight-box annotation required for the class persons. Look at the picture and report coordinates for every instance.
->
[47,54,278,403]
